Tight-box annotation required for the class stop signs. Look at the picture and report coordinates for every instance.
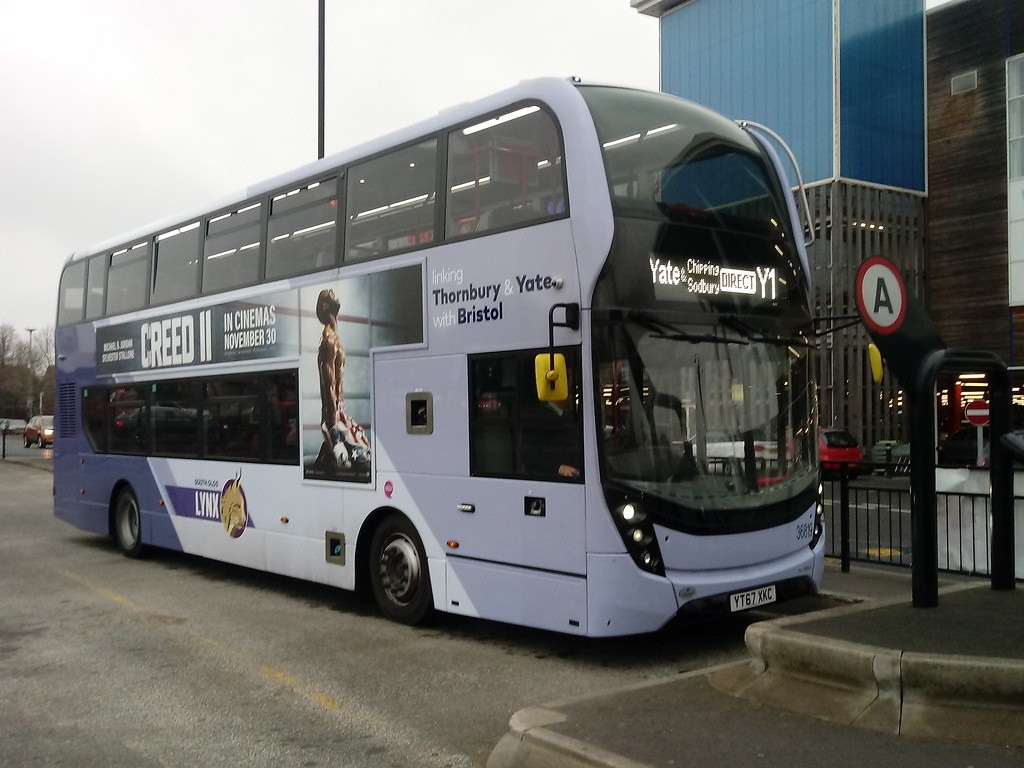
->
[965,400,990,427]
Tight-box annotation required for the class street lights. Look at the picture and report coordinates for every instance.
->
[25,328,36,418]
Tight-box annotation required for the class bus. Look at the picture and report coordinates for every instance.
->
[49,75,829,639]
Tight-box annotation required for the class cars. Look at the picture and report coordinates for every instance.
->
[681,426,782,476]
[785,427,865,477]
[940,427,992,468]
[0,417,9,426]
[4,420,28,435]
[23,415,54,448]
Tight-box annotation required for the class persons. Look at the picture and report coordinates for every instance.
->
[517,360,580,477]
[246,428,262,458]
[314,289,370,473]
